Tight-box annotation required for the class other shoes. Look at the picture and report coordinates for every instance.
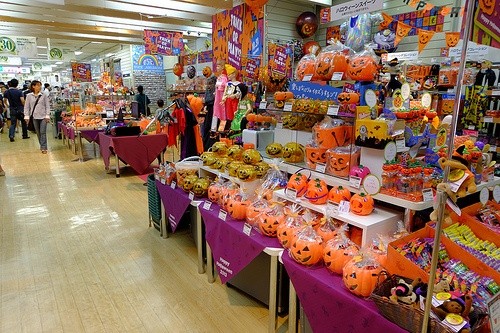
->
[10,138,15,142]
[0,170,5,176]
[40,147,47,154]
[23,136,30,139]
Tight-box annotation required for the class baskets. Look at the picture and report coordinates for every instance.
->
[371,270,490,333]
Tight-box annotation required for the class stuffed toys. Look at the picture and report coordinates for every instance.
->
[430,183,457,225]
[438,156,477,198]
[390,277,473,333]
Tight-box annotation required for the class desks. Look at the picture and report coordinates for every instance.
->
[264,158,500,231]
[198,198,285,333]
[278,251,409,333]
[58,122,168,178]
[157,179,209,274]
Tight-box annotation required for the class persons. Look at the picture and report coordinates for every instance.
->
[0,78,50,154]
[202,59,252,148]
[54,97,72,139]
[0,163,5,175]
[52,86,65,95]
[155,99,174,125]
[133,85,150,117]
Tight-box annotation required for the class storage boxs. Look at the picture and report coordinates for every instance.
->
[242,129,274,150]
[386,198,500,329]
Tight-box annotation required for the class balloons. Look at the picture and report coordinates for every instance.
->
[373,27,398,57]
[203,66,212,78]
[296,11,318,39]
[173,63,183,76]
[187,66,196,79]
[303,42,321,56]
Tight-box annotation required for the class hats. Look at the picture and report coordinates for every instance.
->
[224,64,236,77]
[233,82,248,101]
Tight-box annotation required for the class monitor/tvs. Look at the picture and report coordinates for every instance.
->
[131,101,142,118]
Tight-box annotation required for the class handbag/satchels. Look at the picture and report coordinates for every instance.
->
[16,110,24,120]
[27,115,36,131]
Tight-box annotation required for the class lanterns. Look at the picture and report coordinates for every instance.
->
[265,88,371,185]
[294,52,377,82]
[176,142,388,297]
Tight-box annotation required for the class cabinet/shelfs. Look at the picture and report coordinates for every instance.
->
[273,188,401,249]
[482,90,500,153]
[198,166,262,198]
